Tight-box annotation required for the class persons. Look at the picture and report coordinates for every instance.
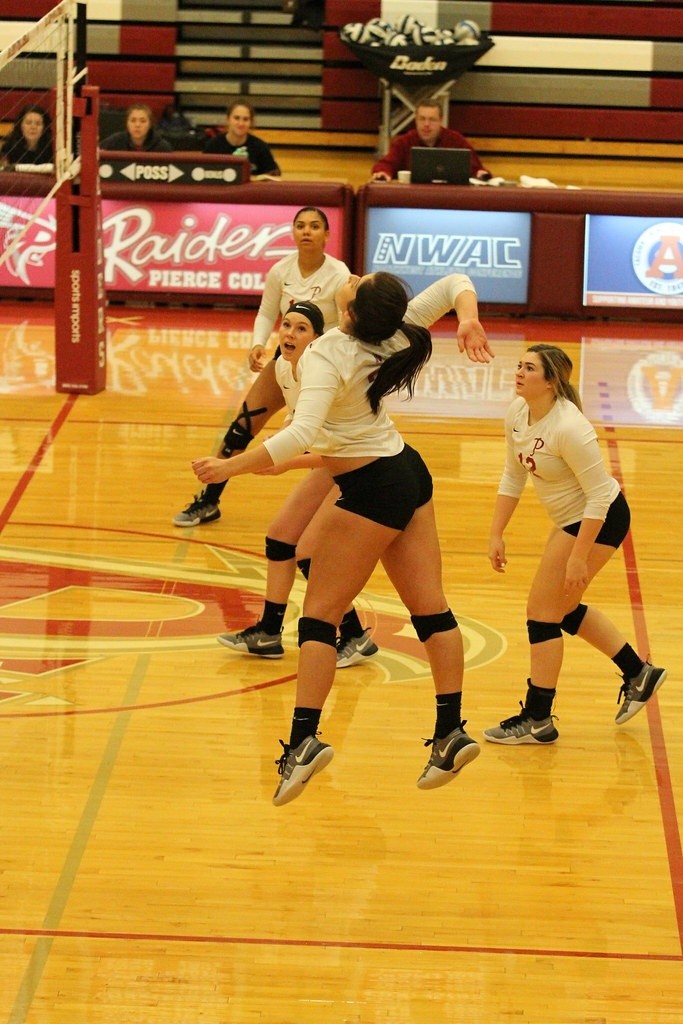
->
[171,208,353,528]
[371,99,491,181]
[216,302,378,670]
[202,99,280,176]
[190,271,496,807]
[0,103,53,164]
[98,105,173,152]
[484,343,668,746]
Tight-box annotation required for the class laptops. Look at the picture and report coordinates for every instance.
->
[411,147,471,185]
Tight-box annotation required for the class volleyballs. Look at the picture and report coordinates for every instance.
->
[338,13,483,48]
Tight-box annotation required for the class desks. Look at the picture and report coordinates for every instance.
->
[355,179,683,321]
[0,169,353,305]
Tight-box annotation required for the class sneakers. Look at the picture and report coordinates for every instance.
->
[172,490,222,528]
[336,626,380,668]
[217,621,285,659]
[272,730,335,807]
[614,653,670,725]
[416,719,481,790]
[483,700,561,745]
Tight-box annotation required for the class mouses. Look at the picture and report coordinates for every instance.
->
[374,175,386,183]
